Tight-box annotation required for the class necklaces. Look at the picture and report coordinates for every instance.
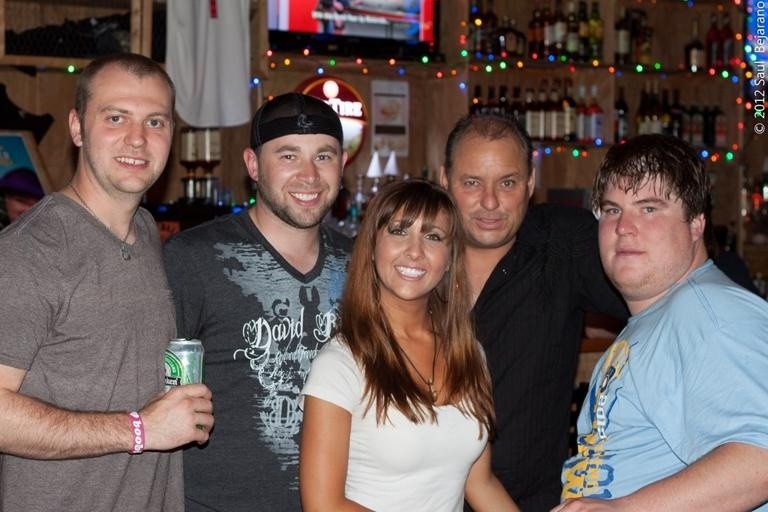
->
[394,310,440,401]
[68,182,134,245]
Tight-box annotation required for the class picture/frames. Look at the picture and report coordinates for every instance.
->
[0,130,57,234]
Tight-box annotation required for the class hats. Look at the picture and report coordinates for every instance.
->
[250,92,343,150]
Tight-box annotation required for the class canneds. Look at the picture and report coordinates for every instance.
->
[163,337,205,430]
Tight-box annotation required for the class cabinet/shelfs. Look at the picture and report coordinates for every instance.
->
[1,2,271,230]
[424,0,744,264]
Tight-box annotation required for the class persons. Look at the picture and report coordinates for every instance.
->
[164,92,358,510]
[550,132,767,512]
[438,109,629,512]
[2,53,215,512]
[299,178,522,511]
[0,168,44,231]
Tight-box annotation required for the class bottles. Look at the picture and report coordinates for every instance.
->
[740,170,768,243]
[323,167,432,241]
[468,0,737,69]
[174,125,236,210]
[754,271,767,298]
[471,77,730,150]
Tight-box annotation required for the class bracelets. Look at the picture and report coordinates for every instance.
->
[127,410,144,456]
